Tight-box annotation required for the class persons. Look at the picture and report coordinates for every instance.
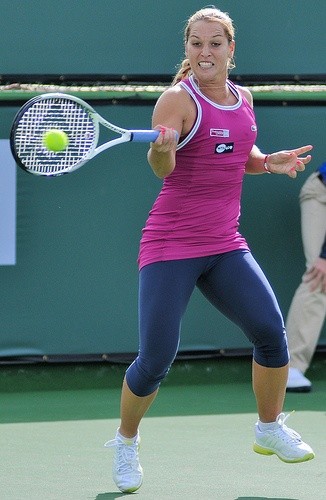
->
[279,160,326,391]
[112,5,316,494]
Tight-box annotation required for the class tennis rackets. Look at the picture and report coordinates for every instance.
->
[9,94,177,177]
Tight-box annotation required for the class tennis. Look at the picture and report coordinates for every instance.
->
[42,131,70,151]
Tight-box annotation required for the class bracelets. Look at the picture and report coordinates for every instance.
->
[263,153,272,176]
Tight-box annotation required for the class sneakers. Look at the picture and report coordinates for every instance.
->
[285,367,313,392]
[111,426,144,493]
[252,417,315,463]
